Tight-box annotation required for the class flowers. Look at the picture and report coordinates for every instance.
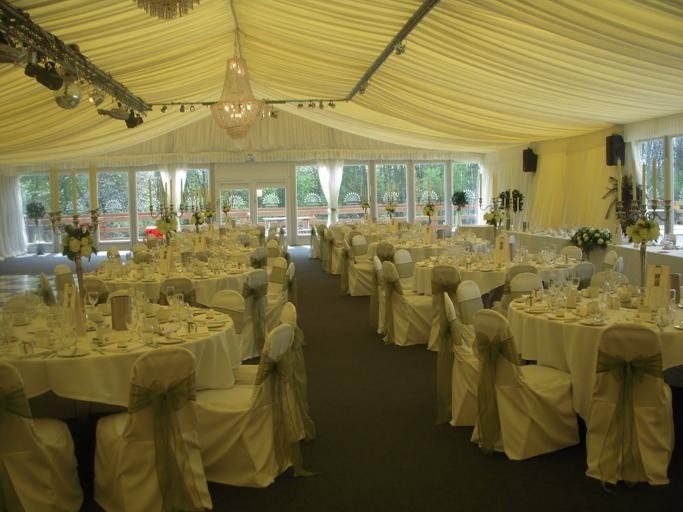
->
[361,199,659,245]
[60,206,232,263]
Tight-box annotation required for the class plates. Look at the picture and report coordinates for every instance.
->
[513,298,683,329]
[13,304,224,357]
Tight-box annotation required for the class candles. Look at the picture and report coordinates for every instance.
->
[417,175,441,197]
[480,170,512,200]
[149,176,233,208]
[50,159,97,215]
[616,157,671,205]
[360,183,371,199]
[381,182,399,197]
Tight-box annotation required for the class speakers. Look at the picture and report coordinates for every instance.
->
[605,133,624,165]
[522,147,536,172]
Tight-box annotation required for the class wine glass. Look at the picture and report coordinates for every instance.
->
[527,268,683,325]
[101,252,241,276]
[0,287,192,344]
[463,245,556,269]
